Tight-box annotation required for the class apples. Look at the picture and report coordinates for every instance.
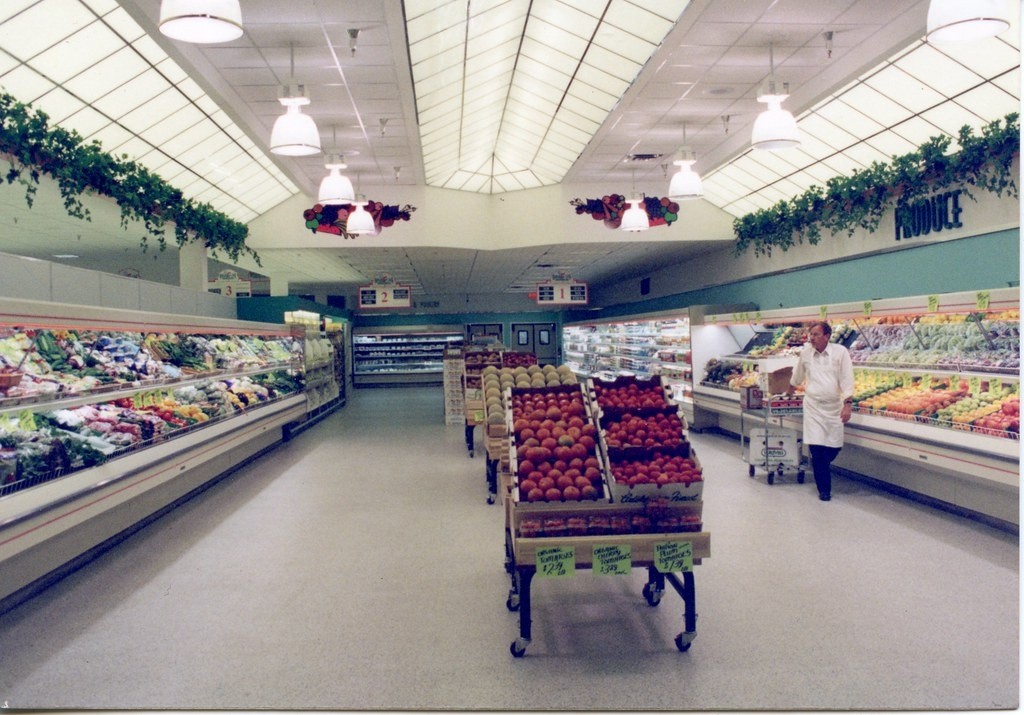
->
[858,386,1020,439]
[511,384,701,500]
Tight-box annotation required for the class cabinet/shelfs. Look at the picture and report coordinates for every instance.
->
[353,325,466,386]
[560,302,1020,535]
[0,291,350,617]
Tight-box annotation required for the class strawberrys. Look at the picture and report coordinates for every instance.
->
[518,503,701,537]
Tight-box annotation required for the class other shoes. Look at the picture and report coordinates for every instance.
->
[820,492,830,501]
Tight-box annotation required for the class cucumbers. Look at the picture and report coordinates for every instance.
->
[66,367,114,385]
[159,340,184,364]
[181,359,209,372]
[31,331,71,372]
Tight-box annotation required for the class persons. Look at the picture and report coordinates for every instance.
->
[786,322,854,501]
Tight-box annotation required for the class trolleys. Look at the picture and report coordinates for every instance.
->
[740,390,811,486]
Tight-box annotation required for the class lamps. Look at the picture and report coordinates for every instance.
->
[345,175,376,234]
[269,44,321,155]
[926,0,1012,46]
[748,49,801,151]
[669,123,704,200]
[621,166,649,232]
[317,126,354,205]
[157,0,246,45]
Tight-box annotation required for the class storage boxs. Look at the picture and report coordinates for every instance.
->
[443,334,707,504]
[749,428,803,466]
[756,365,793,393]
[740,385,762,410]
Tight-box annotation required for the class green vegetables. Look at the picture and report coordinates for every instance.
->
[19,415,104,478]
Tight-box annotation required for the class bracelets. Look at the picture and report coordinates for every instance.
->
[845,401,852,403]
